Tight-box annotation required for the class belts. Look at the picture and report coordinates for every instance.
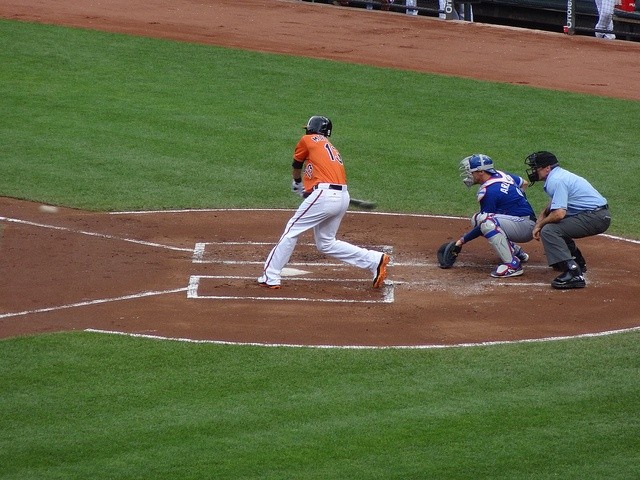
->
[519,216,538,223]
[315,184,342,190]
[590,204,609,214]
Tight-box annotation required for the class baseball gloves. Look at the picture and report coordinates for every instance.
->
[437,241,462,269]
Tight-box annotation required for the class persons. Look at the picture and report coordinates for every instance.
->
[596,0,617,40]
[436,154,537,278]
[531,150,612,289]
[257,116,390,290]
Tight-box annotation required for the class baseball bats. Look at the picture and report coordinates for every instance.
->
[293,188,378,210]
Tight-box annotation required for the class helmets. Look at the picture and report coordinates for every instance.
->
[524,151,560,187]
[458,154,497,189]
[302,116,332,137]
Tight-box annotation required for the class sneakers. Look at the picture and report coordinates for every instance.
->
[373,253,390,288]
[257,276,281,289]
[491,256,524,277]
[553,256,587,273]
[551,271,586,288]
[513,247,529,262]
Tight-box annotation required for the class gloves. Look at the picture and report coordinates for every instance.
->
[299,187,306,197]
[291,179,303,193]
